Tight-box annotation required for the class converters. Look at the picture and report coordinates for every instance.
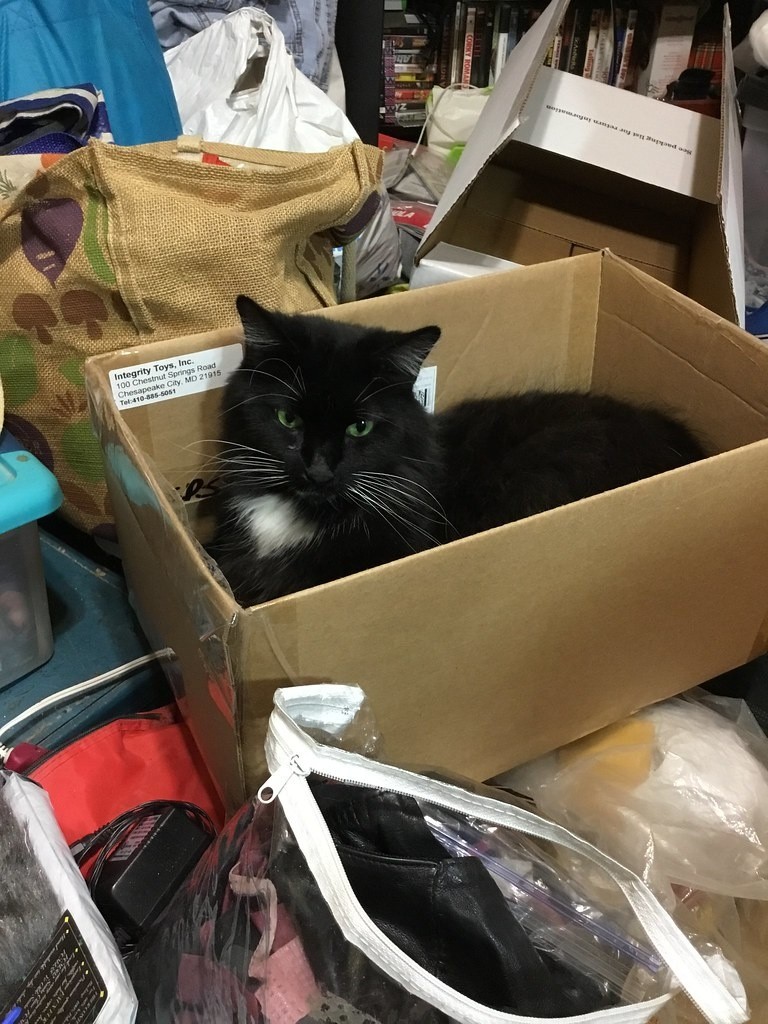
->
[90,807,211,941]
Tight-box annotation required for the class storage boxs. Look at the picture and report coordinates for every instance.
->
[0,428,65,695]
[410,0,768,334]
[80,245,768,822]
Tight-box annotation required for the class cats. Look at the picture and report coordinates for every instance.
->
[178,295,713,611]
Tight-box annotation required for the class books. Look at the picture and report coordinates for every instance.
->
[380,9,439,128]
[544,4,698,101]
[441,2,541,89]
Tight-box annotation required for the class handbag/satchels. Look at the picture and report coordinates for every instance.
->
[20,701,227,882]
[0,133,384,559]
[161,6,362,171]
[123,684,753,1024]
